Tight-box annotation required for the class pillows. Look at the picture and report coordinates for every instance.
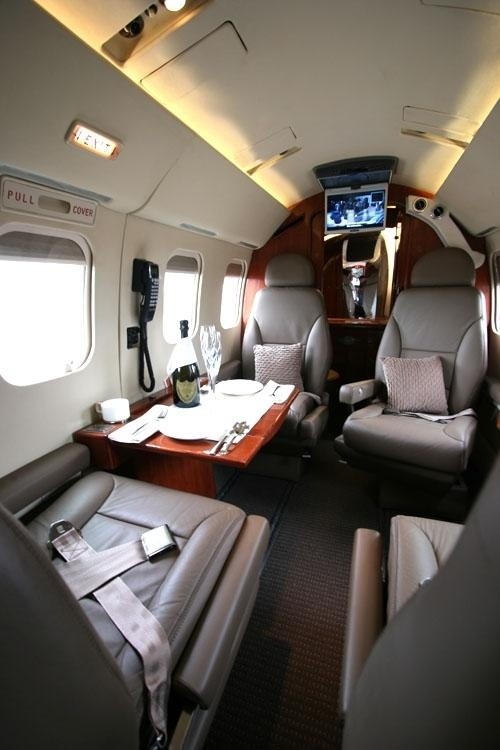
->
[381,356,448,416]
[253,343,304,392]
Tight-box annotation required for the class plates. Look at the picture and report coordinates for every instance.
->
[258,379,296,405]
[157,417,216,441]
[103,403,168,445]
[213,379,264,396]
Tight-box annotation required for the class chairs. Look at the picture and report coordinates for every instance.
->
[339,247,488,506]
[217,254,333,459]
[342,461,500,750]
[0,441,271,750]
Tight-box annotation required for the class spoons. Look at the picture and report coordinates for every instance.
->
[210,421,249,455]
[269,385,281,399]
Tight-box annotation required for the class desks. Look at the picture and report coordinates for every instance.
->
[73,378,300,498]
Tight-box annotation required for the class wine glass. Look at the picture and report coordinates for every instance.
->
[199,320,223,401]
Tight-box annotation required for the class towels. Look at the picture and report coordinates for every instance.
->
[166,337,199,377]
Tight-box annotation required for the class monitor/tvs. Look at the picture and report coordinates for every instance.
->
[324,182,388,235]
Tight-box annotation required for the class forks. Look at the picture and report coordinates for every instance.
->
[130,407,168,436]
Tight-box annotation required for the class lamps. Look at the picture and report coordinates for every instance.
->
[159,0,186,12]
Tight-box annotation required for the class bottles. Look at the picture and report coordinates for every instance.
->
[171,319,200,408]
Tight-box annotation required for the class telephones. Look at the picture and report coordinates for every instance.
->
[132,258,159,323]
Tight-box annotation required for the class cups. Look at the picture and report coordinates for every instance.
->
[95,397,131,423]
